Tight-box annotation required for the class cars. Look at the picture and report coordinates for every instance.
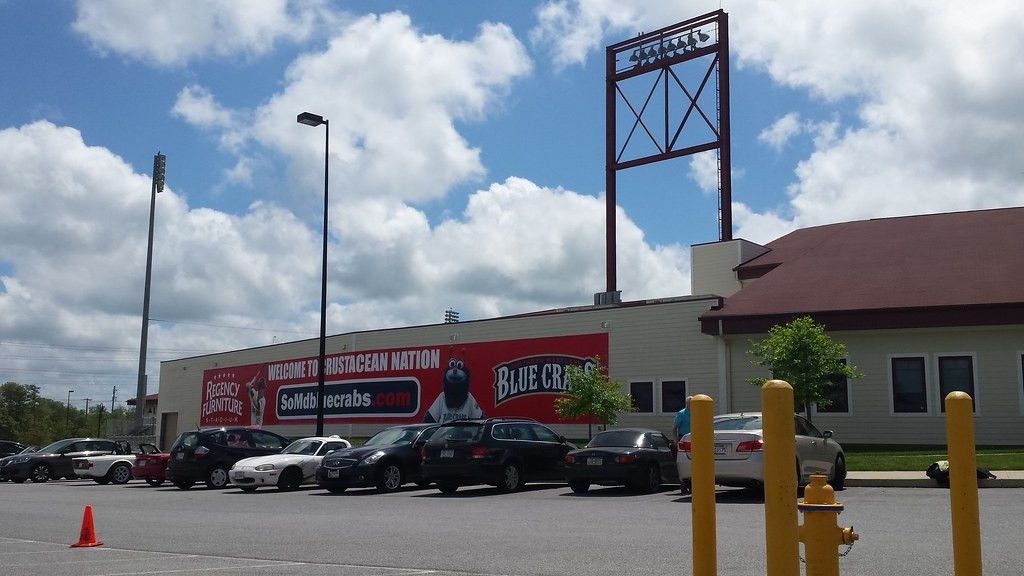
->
[0,436,134,483]
[562,427,678,494]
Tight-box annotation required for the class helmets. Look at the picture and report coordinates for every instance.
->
[258,377,267,388]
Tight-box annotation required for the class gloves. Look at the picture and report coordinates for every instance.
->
[250,389,254,397]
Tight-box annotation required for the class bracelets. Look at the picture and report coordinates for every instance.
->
[249,390,255,398]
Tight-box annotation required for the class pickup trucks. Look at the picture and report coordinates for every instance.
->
[71,439,149,485]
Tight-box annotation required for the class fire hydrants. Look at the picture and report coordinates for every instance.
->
[797,475,860,576]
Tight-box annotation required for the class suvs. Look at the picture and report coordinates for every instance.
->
[419,415,580,494]
[163,426,296,491]
[671,410,847,495]
[316,422,443,493]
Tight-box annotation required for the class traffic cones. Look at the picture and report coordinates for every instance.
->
[70,504,104,548]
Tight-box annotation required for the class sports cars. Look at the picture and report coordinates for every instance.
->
[130,442,171,486]
[228,433,353,492]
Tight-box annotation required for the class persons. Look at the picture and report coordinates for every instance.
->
[672,395,694,494]
[245,378,265,424]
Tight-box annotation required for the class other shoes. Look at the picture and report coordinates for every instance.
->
[682,490,689,494]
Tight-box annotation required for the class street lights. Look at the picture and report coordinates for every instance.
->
[294,110,331,437]
[133,150,167,441]
[66,389,75,429]
